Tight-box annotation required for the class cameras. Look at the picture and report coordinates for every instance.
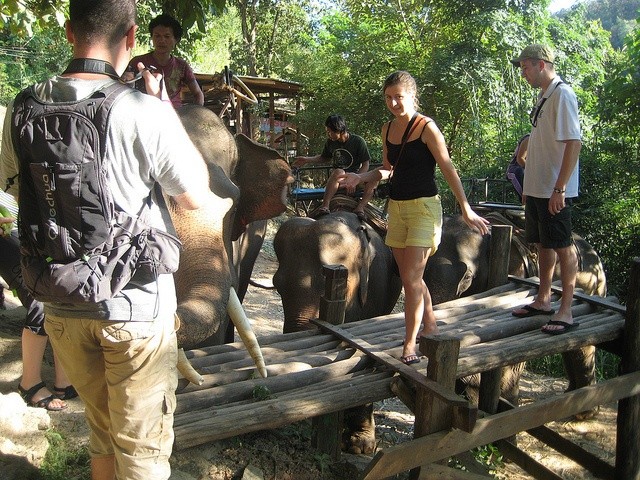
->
[121,67,150,91]
[373,182,393,203]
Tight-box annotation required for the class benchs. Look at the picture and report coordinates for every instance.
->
[292,163,390,220]
[454,177,526,213]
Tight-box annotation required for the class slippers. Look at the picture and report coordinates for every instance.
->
[319,207,330,214]
[402,337,420,345]
[401,355,420,365]
[512,305,554,318]
[542,320,579,335]
[354,211,367,222]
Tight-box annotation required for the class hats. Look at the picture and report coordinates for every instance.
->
[510,44,554,67]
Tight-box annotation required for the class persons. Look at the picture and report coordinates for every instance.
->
[292,115,380,216]
[337,70,490,361]
[0,189,79,411]
[513,44,582,330]
[0,0,209,480]
[127,14,205,105]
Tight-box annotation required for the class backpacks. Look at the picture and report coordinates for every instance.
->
[11,82,182,305]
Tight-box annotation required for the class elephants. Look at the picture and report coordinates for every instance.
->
[272,193,404,455]
[151,102,295,350]
[423,205,611,424]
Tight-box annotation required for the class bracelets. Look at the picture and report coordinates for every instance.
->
[551,188,566,194]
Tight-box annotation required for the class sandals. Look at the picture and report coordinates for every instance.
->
[17,381,65,412]
[52,385,78,400]
